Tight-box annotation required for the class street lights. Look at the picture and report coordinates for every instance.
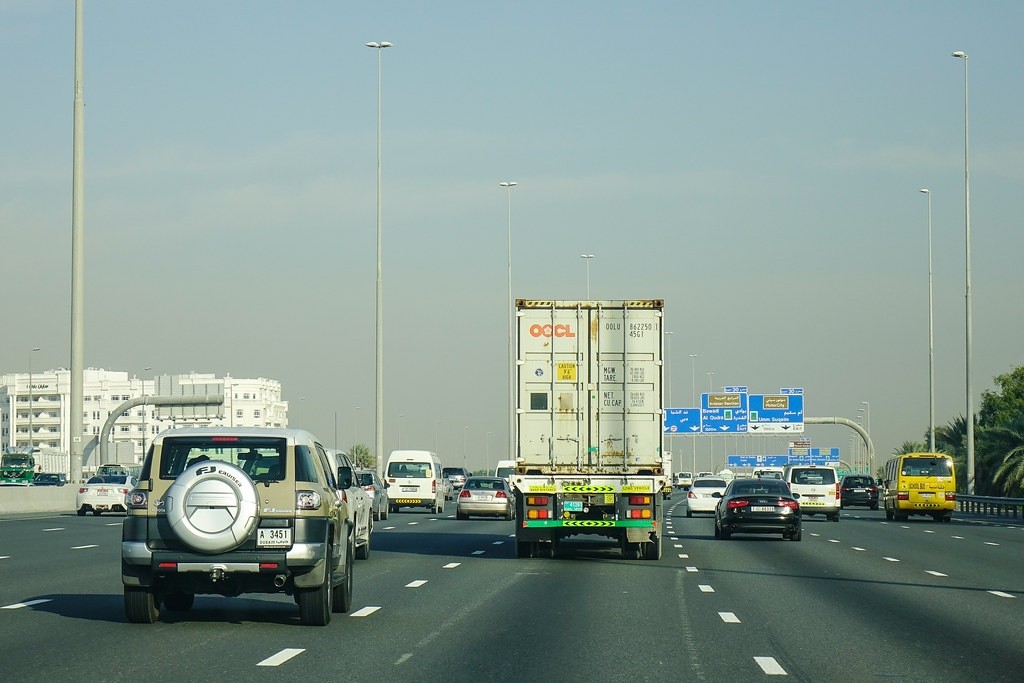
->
[498,182,518,460]
[398,414,404,450]
[353,407,359,466]
[28,347,40,447]
[950,50,975,497]
[298,397,306,428]
[920,188,935,453]
[486,432,493,477]
[435,421,441,455]
[580,254,594,302]
[141,366,152,462]
[230,383,239,463]
[463,427,469,468]
[849,402,870,475]
[364,42,397,484]
[664,330,715,477]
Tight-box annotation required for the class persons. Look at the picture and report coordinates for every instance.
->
[475,480,481,488]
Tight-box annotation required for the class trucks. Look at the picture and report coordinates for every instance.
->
[0,446,68,487]
[513,298,665,561]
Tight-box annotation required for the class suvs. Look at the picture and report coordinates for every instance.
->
[122,425,352,626]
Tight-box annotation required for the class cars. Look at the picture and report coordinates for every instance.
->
[323,448,517,560]
[663,450,881,543]
[75,474,138,516]
[32,472,66,486]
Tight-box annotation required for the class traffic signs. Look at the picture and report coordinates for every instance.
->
[701,392,748,434]
[747,394,804,433]
[663,408,701,434]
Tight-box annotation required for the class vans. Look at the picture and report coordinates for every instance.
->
[877,452,956,524]
[96,463,142,479]
